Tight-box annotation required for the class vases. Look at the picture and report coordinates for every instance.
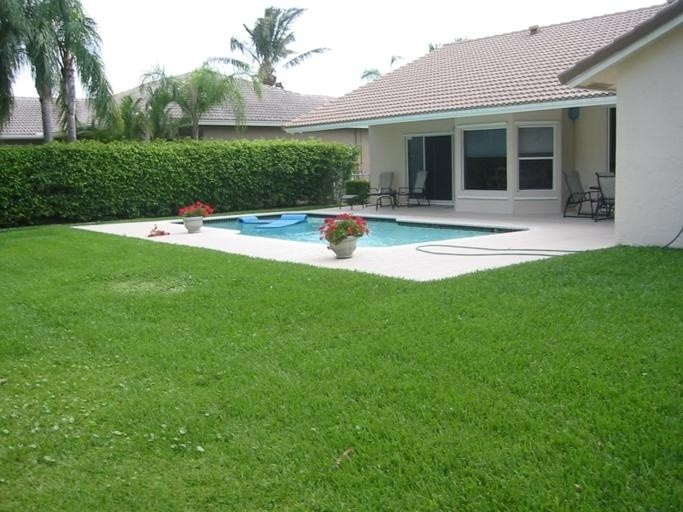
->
[328,233,359,259]
[184,212,204,234]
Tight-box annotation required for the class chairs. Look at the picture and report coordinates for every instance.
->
[562,167,597,220]
[592,172,614,223]
[334,169,432,213]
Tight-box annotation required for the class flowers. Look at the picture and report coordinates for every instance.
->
[316,212,369,239]
[178,202,215,217]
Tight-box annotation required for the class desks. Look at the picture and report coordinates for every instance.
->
[588,184,614,218]
